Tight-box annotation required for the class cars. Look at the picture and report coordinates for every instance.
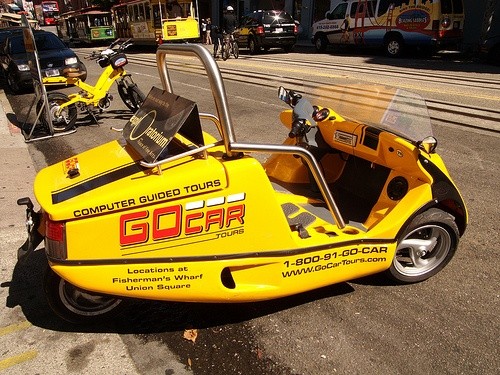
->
[0,30,88,93]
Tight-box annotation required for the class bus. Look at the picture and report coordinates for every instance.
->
[56,7,115,45]
[1,13,39,30]
[112,0,200,47]
[42,1,60,25]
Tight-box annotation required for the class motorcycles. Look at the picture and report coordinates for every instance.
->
[29,41,470,327]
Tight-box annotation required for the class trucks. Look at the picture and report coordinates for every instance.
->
[6,4,21,13]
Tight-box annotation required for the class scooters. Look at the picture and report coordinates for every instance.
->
[36,38,146,133]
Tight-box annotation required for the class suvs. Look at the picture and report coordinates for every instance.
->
[233,10,298,55]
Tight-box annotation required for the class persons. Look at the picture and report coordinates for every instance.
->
[205,17,224,58]
[201,18,212,45]
[220,6,237,57]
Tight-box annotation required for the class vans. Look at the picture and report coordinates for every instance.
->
[310,0,465,59]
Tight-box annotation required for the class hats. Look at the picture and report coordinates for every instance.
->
[227,6,235,10]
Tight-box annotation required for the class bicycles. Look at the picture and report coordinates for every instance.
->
[213,26,239,61]
